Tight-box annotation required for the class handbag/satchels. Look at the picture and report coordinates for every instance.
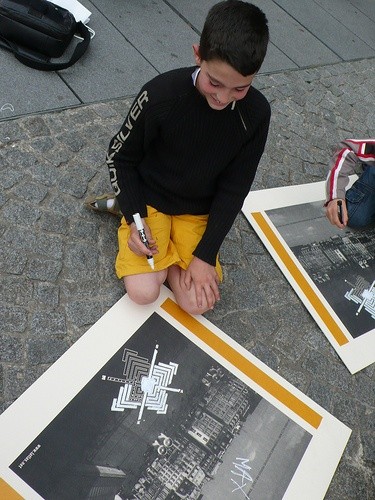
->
[0,0,90,71]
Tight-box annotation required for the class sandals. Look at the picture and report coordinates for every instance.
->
[84,193,123,218]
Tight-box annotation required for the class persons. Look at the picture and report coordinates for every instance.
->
[323,139,375,230]
[84,0,271,315]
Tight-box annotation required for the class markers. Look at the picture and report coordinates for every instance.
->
[133,213,155,270]
[337,200,343,224]
